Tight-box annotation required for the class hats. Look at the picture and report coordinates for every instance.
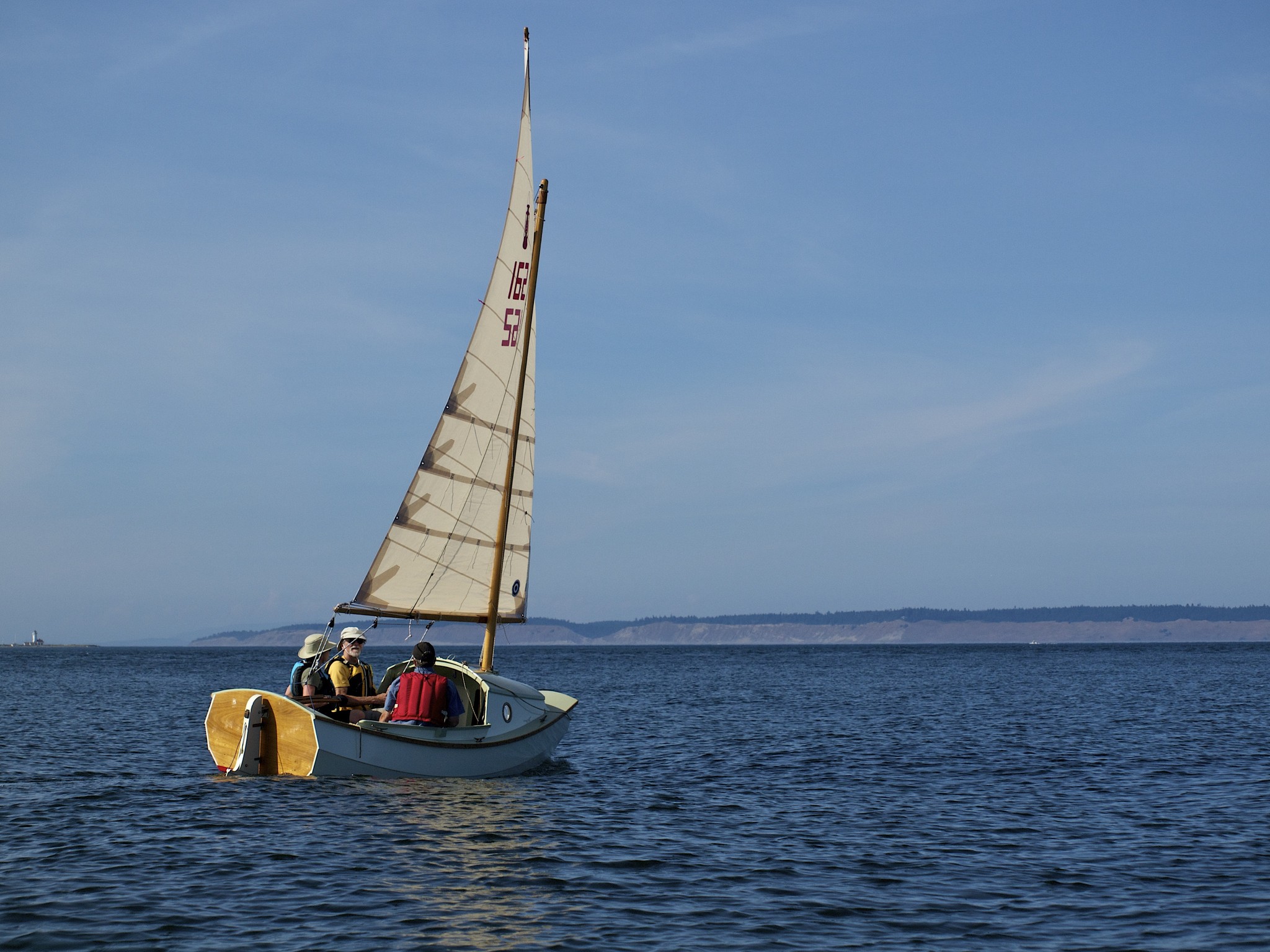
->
[297,633,337,659]
[412,642,436,660]
[337,627,366,650]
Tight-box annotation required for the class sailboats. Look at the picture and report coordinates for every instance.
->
[202,21,579,781]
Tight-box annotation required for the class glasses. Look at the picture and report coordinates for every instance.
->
[346,638,364,644]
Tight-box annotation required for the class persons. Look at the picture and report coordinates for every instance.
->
[324,627,391,724]
[285,633,365,724]
[378,641,465,728]
